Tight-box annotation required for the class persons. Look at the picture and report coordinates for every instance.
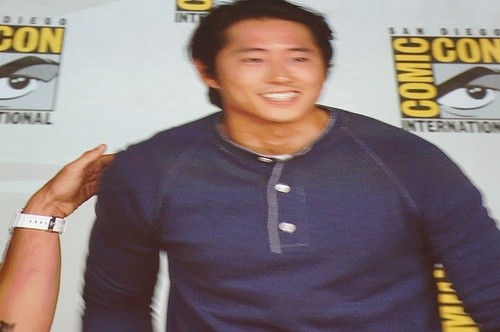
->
[0,143,119,332]
[83,0,500,331]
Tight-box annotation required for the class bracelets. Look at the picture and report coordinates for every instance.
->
[9,210,65,236]
